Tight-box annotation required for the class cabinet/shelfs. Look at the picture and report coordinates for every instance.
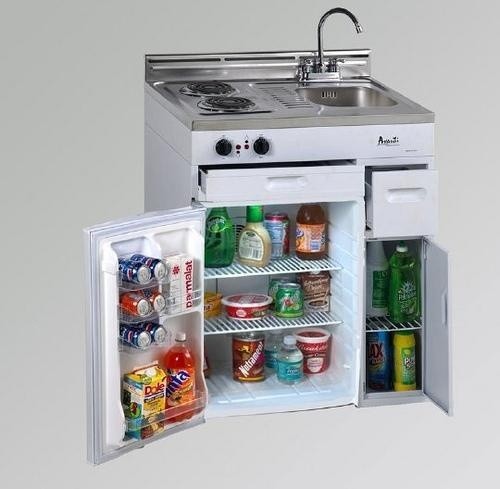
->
[83,160,365,468]
[359,156,455,420]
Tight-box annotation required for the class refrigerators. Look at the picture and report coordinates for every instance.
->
[86,164,472,470]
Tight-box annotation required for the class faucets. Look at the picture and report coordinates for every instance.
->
[312,7,362,73]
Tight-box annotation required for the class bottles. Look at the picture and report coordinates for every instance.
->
[238,205,272,267]
[367,330,391,392]
[389,241,420,324]
[295,202,329,260]
[205,207,236,268]
[366,241,389,317]
[275,335,303,385]
[265,329,283,368]
[393,330,417,392]
[164,332,196,423]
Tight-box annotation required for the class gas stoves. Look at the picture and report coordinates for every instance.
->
[179,76,277,156]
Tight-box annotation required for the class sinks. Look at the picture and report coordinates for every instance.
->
[293,79,400,109]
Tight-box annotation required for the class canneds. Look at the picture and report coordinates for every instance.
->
[138,323,166,344]
[118,258,152,286]
[120,292,152,316]
[274,282,305,321]
[136,287,167,315]
[265,212,290,259]
[131,255,167,282]
[119,323,150,349]
[293,327,331,374]
[268,277,292,315]
[231,331,265,382]
